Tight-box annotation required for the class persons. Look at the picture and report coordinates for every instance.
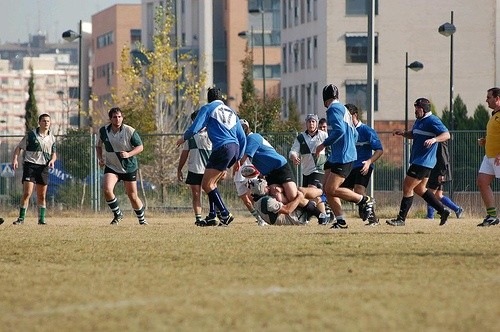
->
[324,104,381,228]
[177,88,247,226]
[477,88,500,228]
[96,107,148,226]
[235,119,328,226]
[13,114,56,226]
[386,98,451,226]
[289,114,328,203]
[316,84,376,229]
[426,141,464,220]
[176,110,213,225]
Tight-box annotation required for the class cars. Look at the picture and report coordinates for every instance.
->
[0,158,156,209]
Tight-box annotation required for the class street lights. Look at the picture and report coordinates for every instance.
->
[238,25,255,107]
[249,8,266,134]
[62,19,83,136]
[403,51,424,176]
[437,10,457,192]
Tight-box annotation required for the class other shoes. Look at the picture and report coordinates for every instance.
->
[0,218,4,225]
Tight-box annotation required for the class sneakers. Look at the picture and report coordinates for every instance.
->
[38,220,47,225]
[317,206,348,229]
[13,218,24,225]
[424,217,434,219]
[363,196,374,221]
[222,213,235,227]
[437,210,450,225]
[195,215,216,226]
[455,207,464,219]
[110,214,124,225]
[141,222,149,225]
[365,219,380,227]
[257,218,269,227]
[195,216,201,225]
[478,215,499,226]
[386,215,405,225]
[217,215,224,226]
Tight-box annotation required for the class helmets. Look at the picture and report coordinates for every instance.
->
[414,98,430,112]
[250,178,268,196]
[305,114,319,123]
[323,84,338,101]
[241,164,259,178]
[208,87,227,105]
[239,119,249,128]
